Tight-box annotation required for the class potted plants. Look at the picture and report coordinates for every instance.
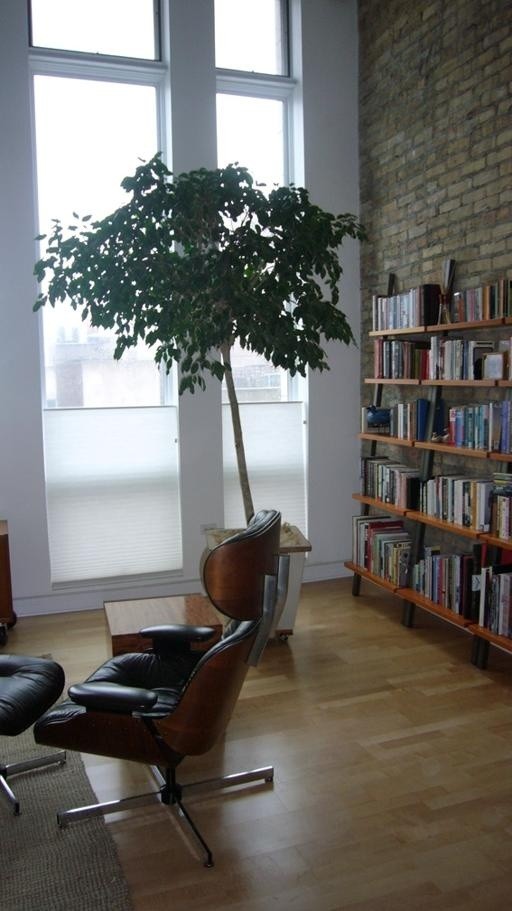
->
[32,150,371,640]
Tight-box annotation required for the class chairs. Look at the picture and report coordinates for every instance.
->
[33,509,274,868]
[0,653,67,814]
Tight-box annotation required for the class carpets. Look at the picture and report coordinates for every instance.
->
[0,697,135,910]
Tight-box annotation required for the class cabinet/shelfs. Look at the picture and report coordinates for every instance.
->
[343,317,512,670]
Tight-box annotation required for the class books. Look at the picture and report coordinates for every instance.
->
[360,457,512,539]
[374,335,512,380]
[352,514,512,637]
[372,279,512,332]
[362,398,512,454]
